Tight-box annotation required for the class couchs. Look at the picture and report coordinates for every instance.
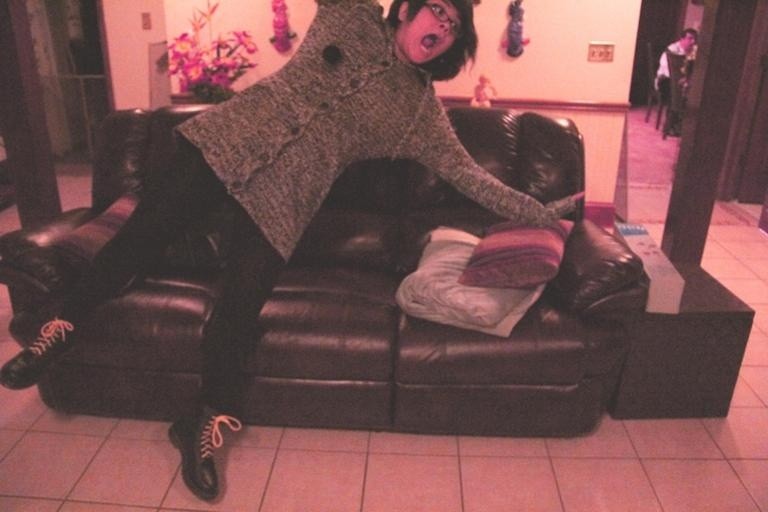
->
[1,108,649,438]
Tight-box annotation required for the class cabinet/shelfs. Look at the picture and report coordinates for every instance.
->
[610,260,755,421]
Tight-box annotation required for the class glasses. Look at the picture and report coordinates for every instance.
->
[425,2,461,36]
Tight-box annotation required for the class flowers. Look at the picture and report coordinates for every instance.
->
[165,1,258,103]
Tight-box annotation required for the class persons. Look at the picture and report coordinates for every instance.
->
[1,1,585,501]
[657,26,700,135]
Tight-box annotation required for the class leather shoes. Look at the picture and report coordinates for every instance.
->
[0,314,78,391]
[168,399,219,502]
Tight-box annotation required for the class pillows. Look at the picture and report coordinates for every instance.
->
[52,191,140,267]
[457,218,574,294]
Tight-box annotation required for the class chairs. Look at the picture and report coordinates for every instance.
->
[662,47,686,140]
[641,42,666,129]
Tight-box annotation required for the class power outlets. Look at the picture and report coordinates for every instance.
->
[142,13,151,29]
